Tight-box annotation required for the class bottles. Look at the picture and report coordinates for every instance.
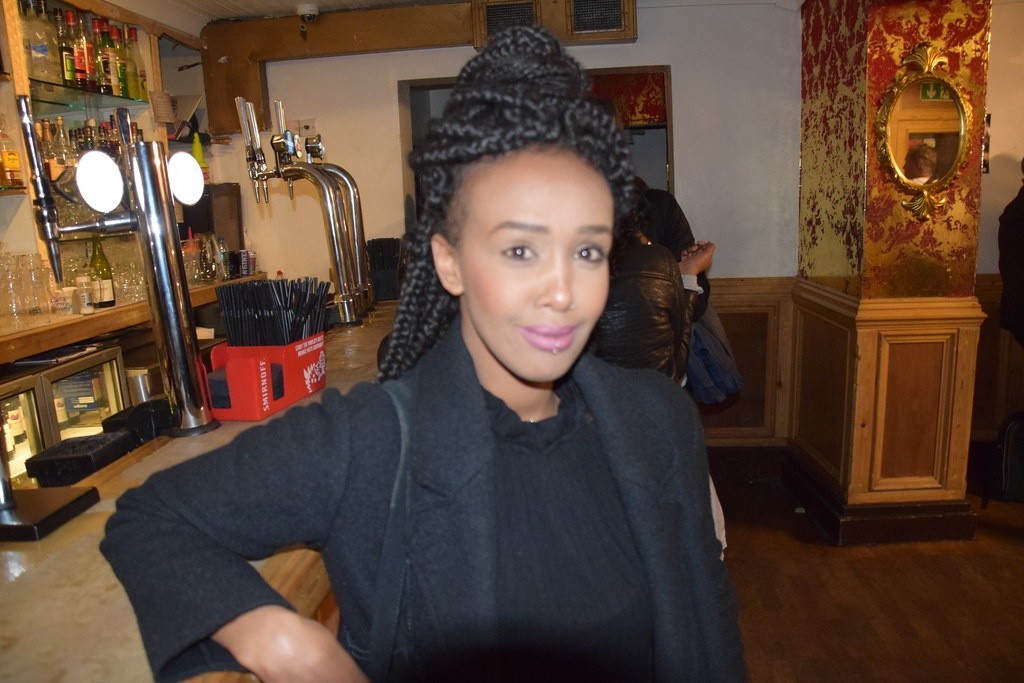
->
[51,384,73,425]
[0,121,24,188]
[93,234,114,311]
[2,403,26,459]
[30,113,144,228]
[18,0,150,102]
[216,238,230,279]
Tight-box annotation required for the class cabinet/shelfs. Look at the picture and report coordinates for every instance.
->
[1,0,267,483]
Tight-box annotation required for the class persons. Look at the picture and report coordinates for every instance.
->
[591,174,733,364]
[594,190,715,383]
[101,26,757,683]
[997,160,1023,344]
[899,144,939,186]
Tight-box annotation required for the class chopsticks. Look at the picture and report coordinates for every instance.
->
[216,276,331,347]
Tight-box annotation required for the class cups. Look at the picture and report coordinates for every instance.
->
[179,234,219,282]
[0,248,61,334]
[64,257,147,316]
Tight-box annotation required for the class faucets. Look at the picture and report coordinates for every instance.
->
[244,159,297,203]
[35,206,65,283]
